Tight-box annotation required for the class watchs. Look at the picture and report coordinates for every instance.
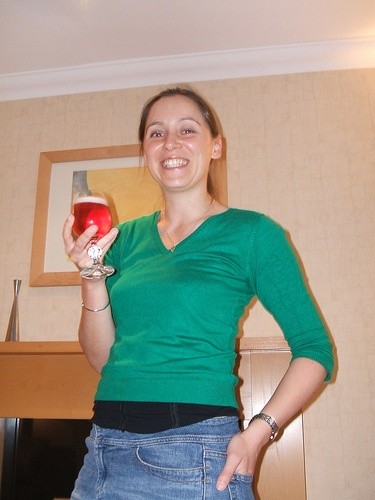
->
[247,413,279,441]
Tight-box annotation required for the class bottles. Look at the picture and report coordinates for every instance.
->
[5,279,22,342]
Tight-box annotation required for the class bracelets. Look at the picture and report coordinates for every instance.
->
[82,302,111,312]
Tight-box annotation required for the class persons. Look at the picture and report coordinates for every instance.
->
[61,86,335,499]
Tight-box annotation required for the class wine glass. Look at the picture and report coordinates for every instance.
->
[72,188,115,280]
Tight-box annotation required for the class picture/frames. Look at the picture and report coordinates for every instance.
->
[29,137,230,286]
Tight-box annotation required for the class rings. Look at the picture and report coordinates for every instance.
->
[88,246,103,259]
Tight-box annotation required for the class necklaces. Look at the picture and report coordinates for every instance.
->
[160,211,201,252]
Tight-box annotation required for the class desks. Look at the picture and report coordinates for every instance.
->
[0,336,305,500]
